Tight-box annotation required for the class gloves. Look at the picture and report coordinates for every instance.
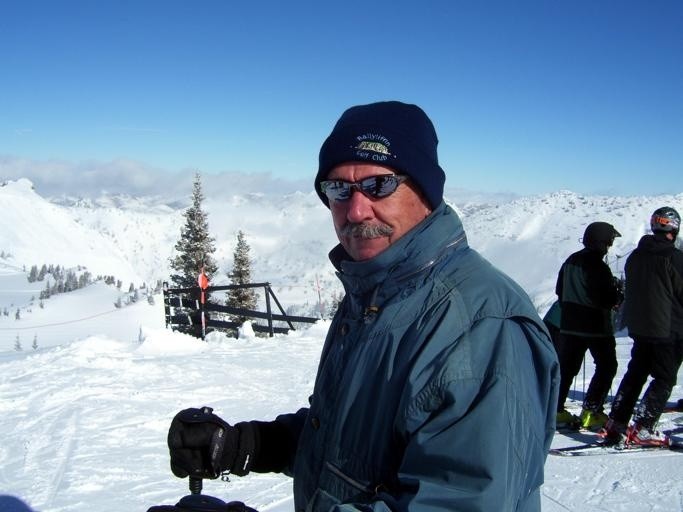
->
[168,408,256,477]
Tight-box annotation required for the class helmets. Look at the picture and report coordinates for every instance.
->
[651,207,681,237]
[582,222,621,255]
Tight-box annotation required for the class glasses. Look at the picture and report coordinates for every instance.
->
[319,173,410,204]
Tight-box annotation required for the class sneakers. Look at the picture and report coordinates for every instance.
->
[556,408,674,447]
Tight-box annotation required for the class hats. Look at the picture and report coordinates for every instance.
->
[314,100,445,210]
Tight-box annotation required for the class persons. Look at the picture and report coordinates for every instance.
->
[542,299,561,347]
[595,207,682,449]
[553,221,623,431]
[167,99,563,512]
